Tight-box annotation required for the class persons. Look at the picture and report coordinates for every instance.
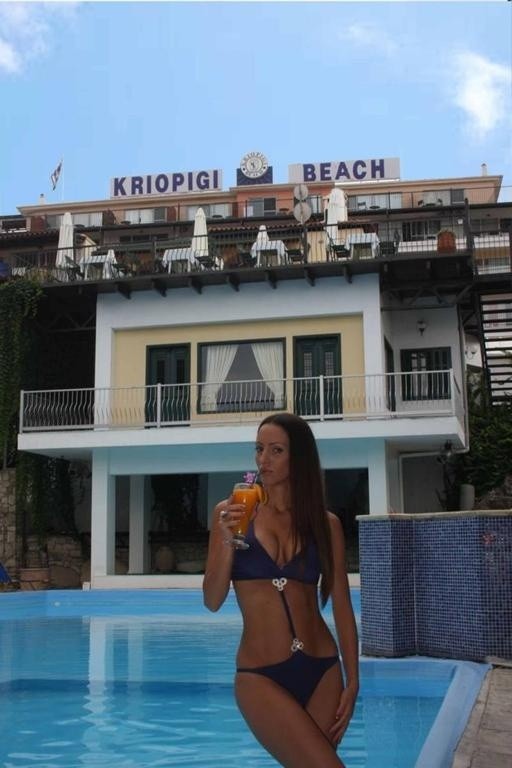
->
[199,412,360,768]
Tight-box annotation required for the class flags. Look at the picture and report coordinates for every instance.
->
[51,157,63,188]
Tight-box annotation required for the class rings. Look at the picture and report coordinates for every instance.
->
[219,510,227,521]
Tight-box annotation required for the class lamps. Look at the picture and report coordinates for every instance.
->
[417,318,428,335]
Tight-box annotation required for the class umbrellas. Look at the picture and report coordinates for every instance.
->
[192,208,209,257]
[250,225,272,268]
[324,189,351,261]
[55,213,77,268]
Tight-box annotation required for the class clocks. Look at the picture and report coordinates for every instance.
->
[241,151,268,179]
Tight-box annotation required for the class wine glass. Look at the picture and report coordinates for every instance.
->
[228,481,258,549]
